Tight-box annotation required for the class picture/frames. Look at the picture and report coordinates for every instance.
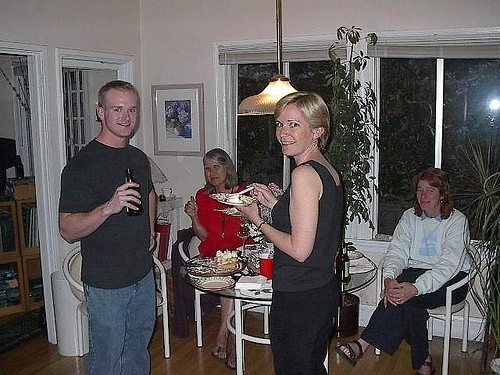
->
[151,83,206,157]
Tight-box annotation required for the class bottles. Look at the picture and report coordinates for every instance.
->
[337,240,351,283]
[125,168,141,216]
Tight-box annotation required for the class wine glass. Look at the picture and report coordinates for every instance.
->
[236,225,250,257]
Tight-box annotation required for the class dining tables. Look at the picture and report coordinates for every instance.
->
[180,244,378,375]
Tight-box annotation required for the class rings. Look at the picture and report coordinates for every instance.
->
[398,299,401,303]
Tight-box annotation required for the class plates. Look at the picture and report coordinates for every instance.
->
[194,276,236,291]
[210,193,254,205]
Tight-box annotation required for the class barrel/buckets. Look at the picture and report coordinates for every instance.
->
[258,256,273,280]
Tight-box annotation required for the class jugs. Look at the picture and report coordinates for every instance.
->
[161,188,172,201]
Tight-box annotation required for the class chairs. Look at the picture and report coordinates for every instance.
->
[178,234,269,347]
[62,245,170,358]
[376,244,481,375]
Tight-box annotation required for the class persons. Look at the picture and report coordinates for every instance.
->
[185,148,257,368]
[237,91,346,375]
[57,81,157,375]
[334,169,471,375]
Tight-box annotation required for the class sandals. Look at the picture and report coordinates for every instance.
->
[415,354,433,375]
[335,340,364,367]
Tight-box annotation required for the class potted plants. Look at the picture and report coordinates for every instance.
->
[445,134,500,375]
[313,22,385,339]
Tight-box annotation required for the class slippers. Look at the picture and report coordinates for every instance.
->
[225,353,236,370]
[211,344,226,361]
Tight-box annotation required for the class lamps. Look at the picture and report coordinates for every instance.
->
[236,0,297,115]
[149,157,168,184]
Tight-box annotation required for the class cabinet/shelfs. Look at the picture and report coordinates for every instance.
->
[0,177,45,317]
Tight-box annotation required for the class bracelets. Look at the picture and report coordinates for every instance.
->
[258,220,266,230]
